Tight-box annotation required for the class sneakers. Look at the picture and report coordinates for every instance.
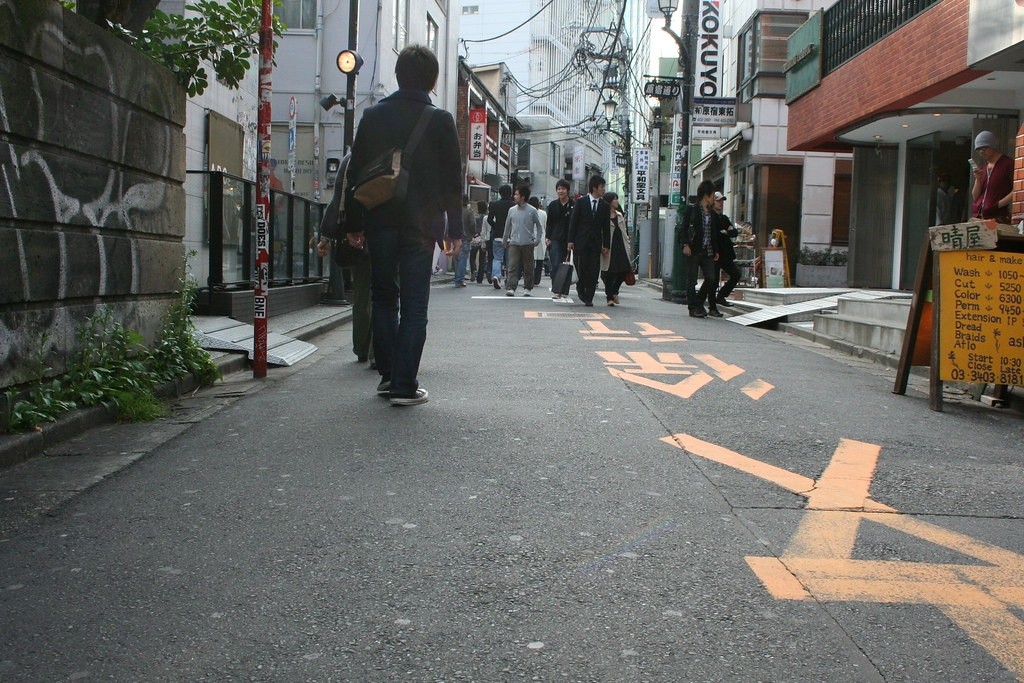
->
[376,373,392,393]
[389,388,429,405]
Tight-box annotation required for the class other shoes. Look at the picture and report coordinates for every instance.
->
[607,294,619,306]
[486,272,493,284]
[584,298,594,306]
[493,276,501,289]
[356,350,374,362]
[523,288,531,296]
[534,279,542,287]
[545,272,550,276]
[506,288,515,296]
[552,291,561,299]
[689,297,734,318]
[455,282,466,287]
[476,277,483,283]
[576,287,588,303]
[470,271,477,282]
[369,354,378,369]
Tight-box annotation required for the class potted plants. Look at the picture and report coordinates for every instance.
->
[795,245,849,288]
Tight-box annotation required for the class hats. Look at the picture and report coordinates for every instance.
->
[714,191,726,201]
[974,131,997,151]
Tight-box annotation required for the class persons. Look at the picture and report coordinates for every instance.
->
[431,194,495,287]
[528,196,547,286]
[503,185,542,296]
[567,175,610,307]
[600,192,631,307]
[488,184,516,288]
[544,179,576,300]
[677,180,741,318]
[346,44,464,405]
[936,172,954,226]
[318,152,376,368]
[971,130,1014,223]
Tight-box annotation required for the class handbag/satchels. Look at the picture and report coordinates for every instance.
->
[550,249,579,296]
[686,204,696,244]
[333,228,371,268]
[431,240,447,275]
[351,146,406,207]
[625,268,637,285]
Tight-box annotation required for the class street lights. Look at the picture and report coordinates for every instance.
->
[655,1,695,304]
[602,93,632,236]
[320,0,366,290]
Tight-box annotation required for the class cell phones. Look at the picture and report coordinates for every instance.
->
[968,159,977,169]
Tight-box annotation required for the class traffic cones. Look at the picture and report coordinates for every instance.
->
[319,258,351,306]
